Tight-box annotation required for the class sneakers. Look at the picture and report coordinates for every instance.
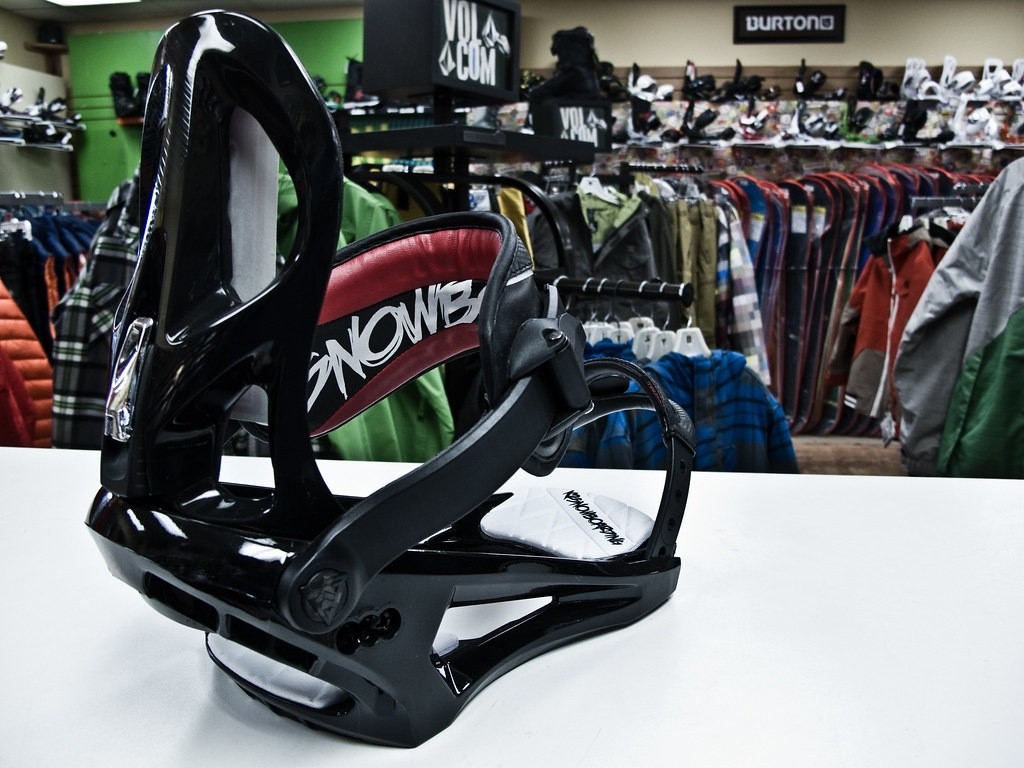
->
[342,89,381,109]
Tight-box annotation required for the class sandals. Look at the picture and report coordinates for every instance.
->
[0,87,86,152]
[502,46,1024,150]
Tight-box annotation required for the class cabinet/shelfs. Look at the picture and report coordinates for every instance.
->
[611,94,1024,150]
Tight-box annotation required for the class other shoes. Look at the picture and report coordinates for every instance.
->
[315,77,341,114]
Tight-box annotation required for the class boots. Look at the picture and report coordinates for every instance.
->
[109,71,149,117]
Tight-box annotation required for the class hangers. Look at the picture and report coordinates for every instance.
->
[542,160,723,362]
[1,190,98,242]
[897,181,990,232]
